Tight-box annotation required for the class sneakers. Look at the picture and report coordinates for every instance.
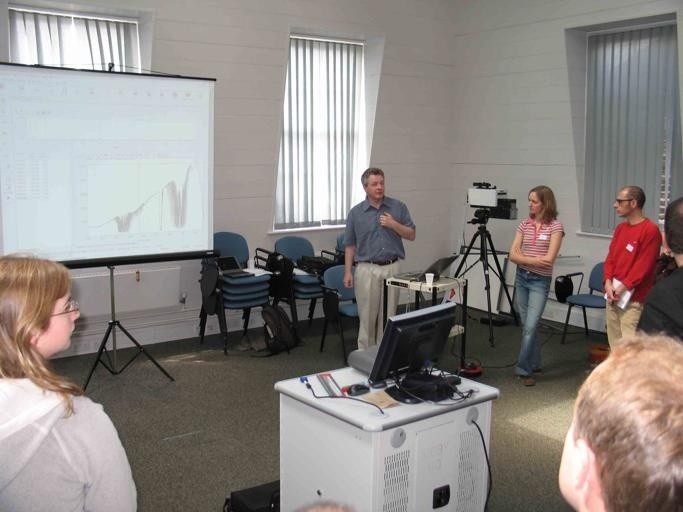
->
[516,374,537,387]
[532,367,545,376]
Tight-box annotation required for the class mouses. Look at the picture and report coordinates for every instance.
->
[347,384,370,396]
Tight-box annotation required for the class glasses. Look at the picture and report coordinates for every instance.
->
[48,299,81,318]
[616,198,634,206]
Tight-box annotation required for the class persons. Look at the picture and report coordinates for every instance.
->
[635,197,683,340]
[507,184,564,386]
[558,330,683,511]
[343,166,417,349]
[602,184,665,351]
[0,255,139,512]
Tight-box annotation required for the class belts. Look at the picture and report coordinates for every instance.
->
[362,257,399,266]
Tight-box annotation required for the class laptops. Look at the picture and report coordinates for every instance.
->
[393,255,460,282]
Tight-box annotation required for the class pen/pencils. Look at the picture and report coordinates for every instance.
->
[612,291,615,305]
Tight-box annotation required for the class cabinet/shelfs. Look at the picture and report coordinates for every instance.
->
[382,268,469,371]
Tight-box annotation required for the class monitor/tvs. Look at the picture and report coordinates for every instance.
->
[368,301,457,402]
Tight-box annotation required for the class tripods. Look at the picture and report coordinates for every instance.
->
[454,226,520,348]
[82,271,175,393]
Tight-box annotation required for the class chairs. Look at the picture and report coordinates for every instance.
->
[196,231,379,366]
[560,261,607,343]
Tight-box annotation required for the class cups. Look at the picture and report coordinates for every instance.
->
[424,272,434,286]
